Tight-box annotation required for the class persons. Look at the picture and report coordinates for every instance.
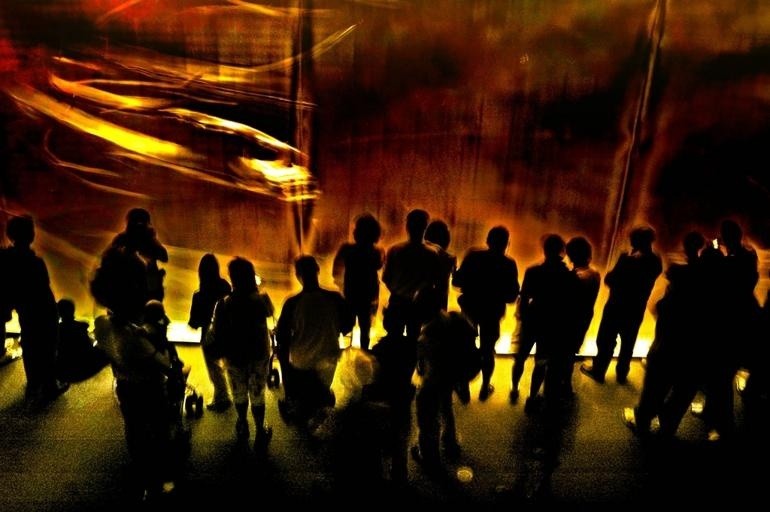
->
[1,208,769,476]
